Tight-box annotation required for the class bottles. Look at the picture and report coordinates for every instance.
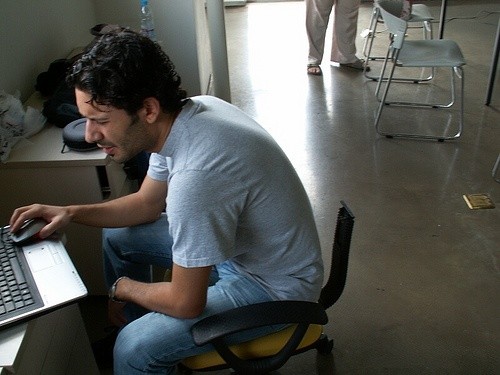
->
[136,0,155,40]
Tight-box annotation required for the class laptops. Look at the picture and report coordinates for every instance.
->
[0,220,87,332]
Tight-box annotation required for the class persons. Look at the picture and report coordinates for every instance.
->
[305,0,370,75]
[10,26,325,375]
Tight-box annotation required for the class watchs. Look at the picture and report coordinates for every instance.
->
[107,277,131,304]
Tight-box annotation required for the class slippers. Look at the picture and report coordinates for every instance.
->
[340,59,369,72]
[307,64,322,75]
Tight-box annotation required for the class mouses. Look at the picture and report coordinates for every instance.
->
[8,217,49,245]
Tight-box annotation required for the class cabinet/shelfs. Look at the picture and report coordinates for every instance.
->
[0,232,99,375]
[0,45,138,297]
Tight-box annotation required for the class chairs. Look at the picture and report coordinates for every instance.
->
[364,0,434,84]
[373,0,468,143]
[161,200,356,375]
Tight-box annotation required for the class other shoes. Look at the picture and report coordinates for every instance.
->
[91,326,119,368]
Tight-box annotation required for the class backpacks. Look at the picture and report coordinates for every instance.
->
[34,53,82,128]
